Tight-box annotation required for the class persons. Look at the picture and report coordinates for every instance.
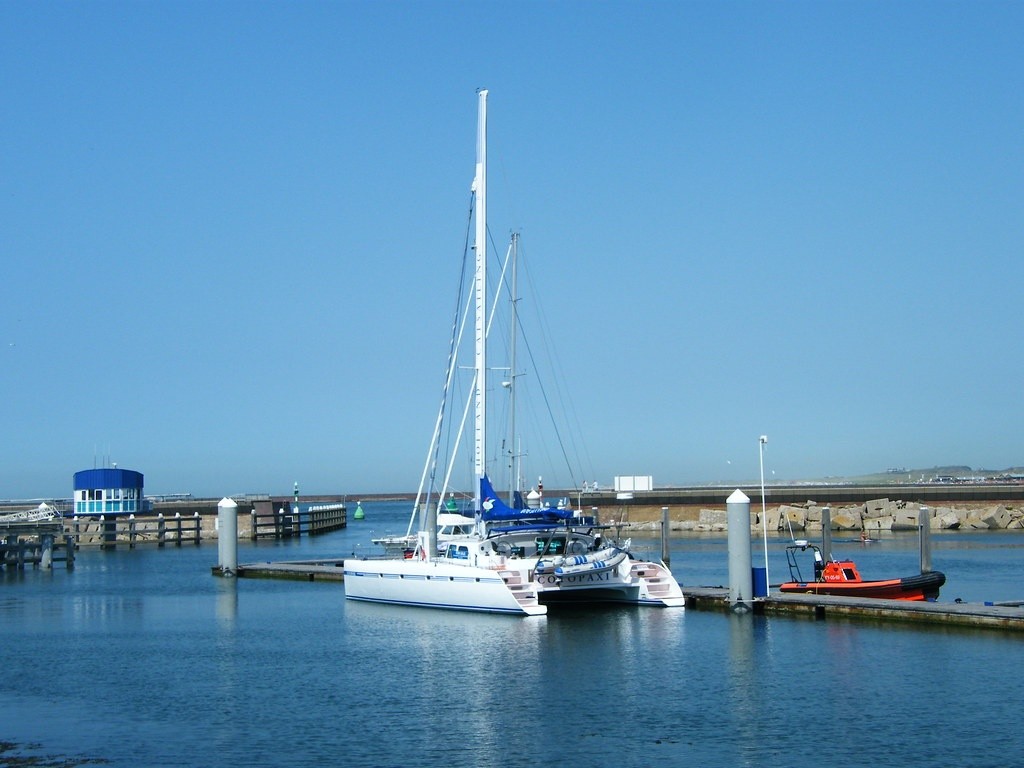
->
[583,480,588,492]
[860,530,870,548]
[592,480,598,492]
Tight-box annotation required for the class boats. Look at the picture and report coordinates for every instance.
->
[779,513,946,602]
[891,523,918,531]
[536,538,631,578]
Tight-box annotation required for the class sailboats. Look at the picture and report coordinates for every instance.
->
[342,86,687,616]
[371,235,595,556]
